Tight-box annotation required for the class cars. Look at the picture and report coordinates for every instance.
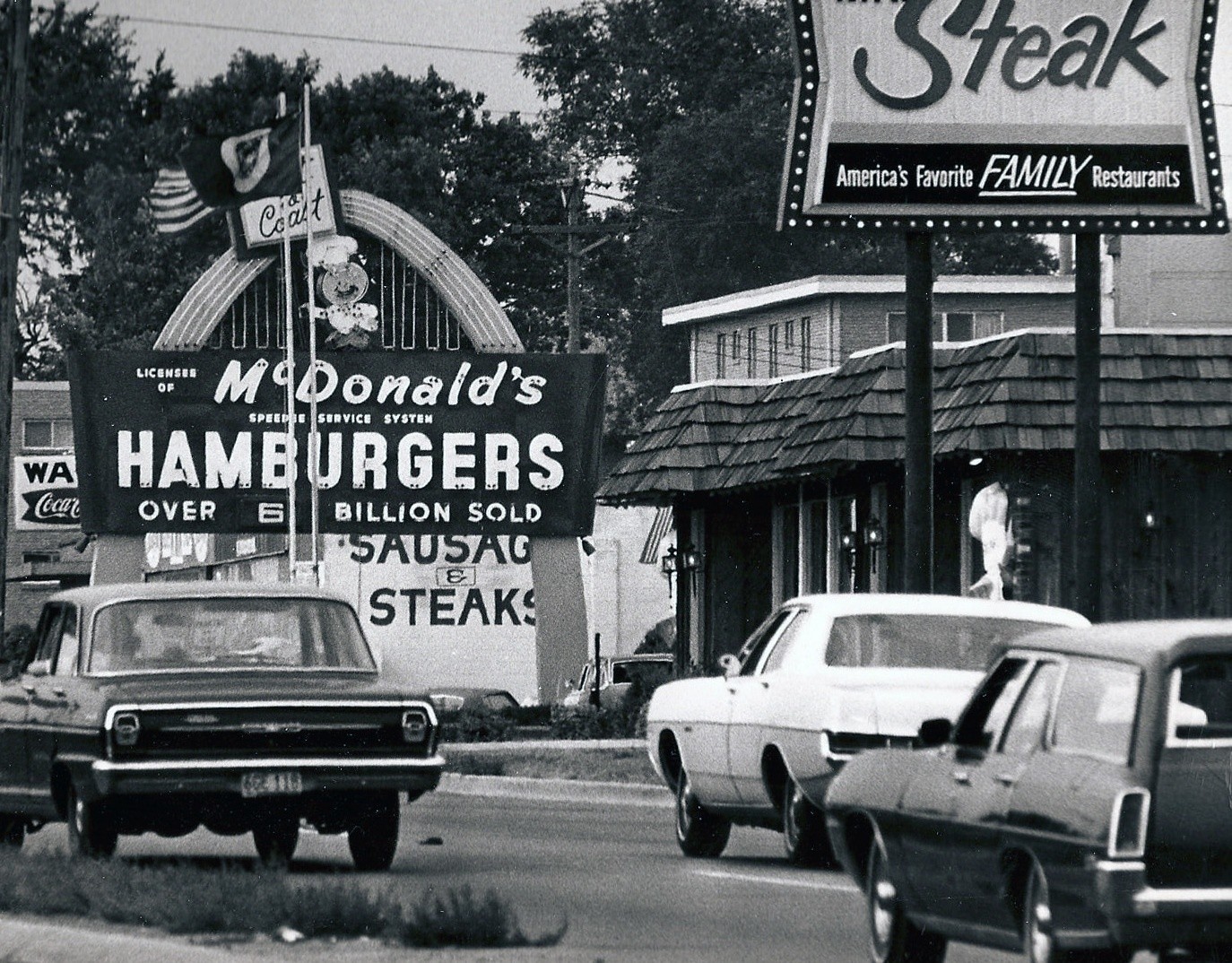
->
[0,577,445,872]
[426,685,521,731]
[644,590,1093,870]
[823,614,1231,963]
[562,652,676,715]
